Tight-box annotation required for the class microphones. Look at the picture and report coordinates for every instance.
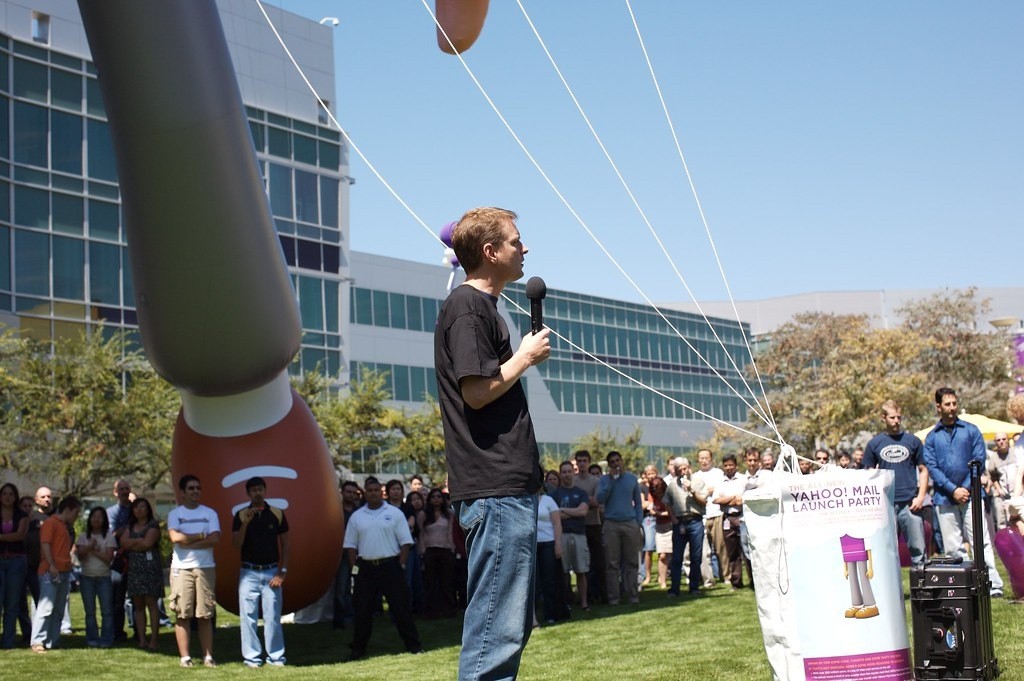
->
[526,277,547,336]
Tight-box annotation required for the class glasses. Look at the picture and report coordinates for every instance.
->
[187,485,202,490]
[817,457,827,460]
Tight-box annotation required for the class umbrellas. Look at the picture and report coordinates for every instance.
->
[913,406,1024,445]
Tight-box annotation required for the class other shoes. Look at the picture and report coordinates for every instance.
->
[204,659,219,668]
[532,577,754,628]
[991,586,1002,597]
[32,645,48,655]
[181,657,193,667]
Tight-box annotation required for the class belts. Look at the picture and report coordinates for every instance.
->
[359,559,398,566]
[241,563,279,573]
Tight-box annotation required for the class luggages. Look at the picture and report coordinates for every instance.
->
[909,460,999,681]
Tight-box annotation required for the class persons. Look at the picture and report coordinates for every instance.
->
[231,475,291,669]
[923,386,1004,598]
[167,473,221,669]
[1,390,1024,629]
[435,209,551,678]
